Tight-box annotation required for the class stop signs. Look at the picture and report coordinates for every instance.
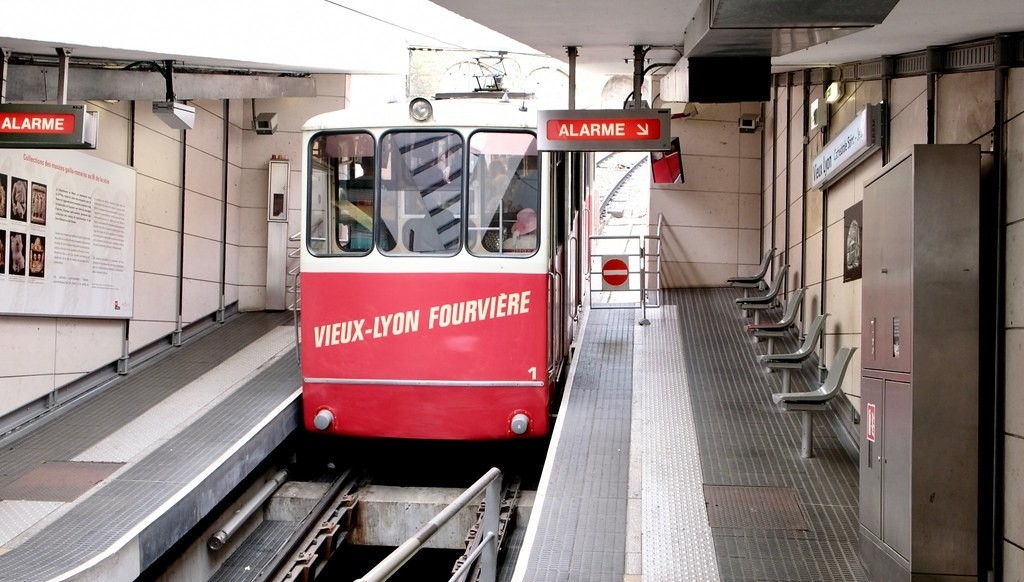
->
[601,259,631,289]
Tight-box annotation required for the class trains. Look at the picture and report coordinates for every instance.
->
[287,57,597,442]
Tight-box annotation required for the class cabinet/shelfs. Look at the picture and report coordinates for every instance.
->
[861,143,979,582]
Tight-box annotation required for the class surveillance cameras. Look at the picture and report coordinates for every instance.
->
[739,118,756,129]
[255,113,277,131]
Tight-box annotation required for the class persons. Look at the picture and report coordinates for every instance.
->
[502,207,537,249]
[340,154,393,207]
[413,144,538,212]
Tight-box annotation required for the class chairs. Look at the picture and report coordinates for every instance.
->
[728,248,857,457]
